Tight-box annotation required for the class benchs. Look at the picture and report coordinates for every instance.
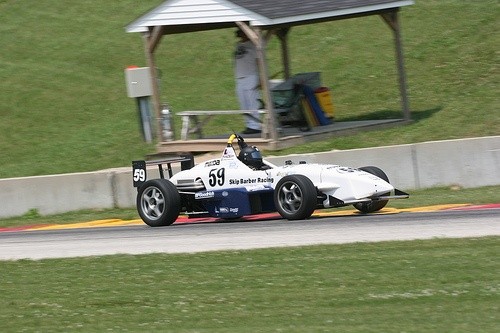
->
[175,109,287,140]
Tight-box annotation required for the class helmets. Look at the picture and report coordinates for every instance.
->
[239,145,262,168]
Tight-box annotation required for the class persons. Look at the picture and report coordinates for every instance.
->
[238,145,263,171]
[232,27,262,134]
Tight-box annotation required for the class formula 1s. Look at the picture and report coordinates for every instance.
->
[132,133,411,226]
